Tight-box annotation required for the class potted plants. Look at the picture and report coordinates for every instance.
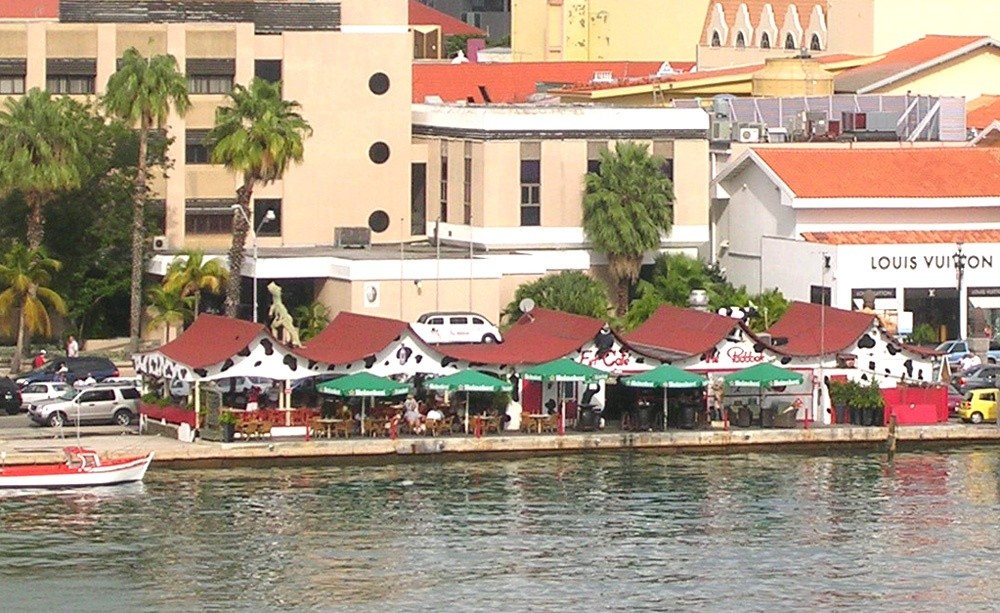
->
[216,412,235,443]
[830,379,886,426]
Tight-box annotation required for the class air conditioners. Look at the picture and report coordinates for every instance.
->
[738,129,761,142]
[153,235,169,250]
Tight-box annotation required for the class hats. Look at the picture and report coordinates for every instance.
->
[40,350,46,354]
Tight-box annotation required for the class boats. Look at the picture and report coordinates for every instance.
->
[0,445,155,489]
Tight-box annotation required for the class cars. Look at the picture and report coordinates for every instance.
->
[957,388,1000,425]
[933,339,1000,395]
[102,377,145,397]
[0,376,23,416]
[947,384,963,417]
[18,382,73,409]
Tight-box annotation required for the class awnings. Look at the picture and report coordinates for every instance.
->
[968,296,1000,309]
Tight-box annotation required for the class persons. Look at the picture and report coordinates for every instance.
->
[959,348,981,370]
[317,394,499,438]
[64,335,79,358]
[35,349,46,367]
[982,320,992,338]
[268,382,278,408]
[243,386,262,411]
[55,362,68,374]
[73,372,96,386]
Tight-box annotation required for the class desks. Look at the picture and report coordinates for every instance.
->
[316,418,342,439]
[529,414,548,434]
[475,416,494,436]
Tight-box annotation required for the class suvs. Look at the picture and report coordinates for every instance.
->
[12,356,120,386]
[27,381,143,428]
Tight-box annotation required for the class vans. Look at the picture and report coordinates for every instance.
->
[415,310,505,345]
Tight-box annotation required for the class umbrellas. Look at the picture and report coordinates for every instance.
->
[725,363,804,406]
[421,368,513,434]
[519,359,608,432]
[622,363,706,429]
[315,372,411,435]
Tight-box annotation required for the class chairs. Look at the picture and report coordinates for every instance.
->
[237,400,559,440]
[728,401,798,429]
